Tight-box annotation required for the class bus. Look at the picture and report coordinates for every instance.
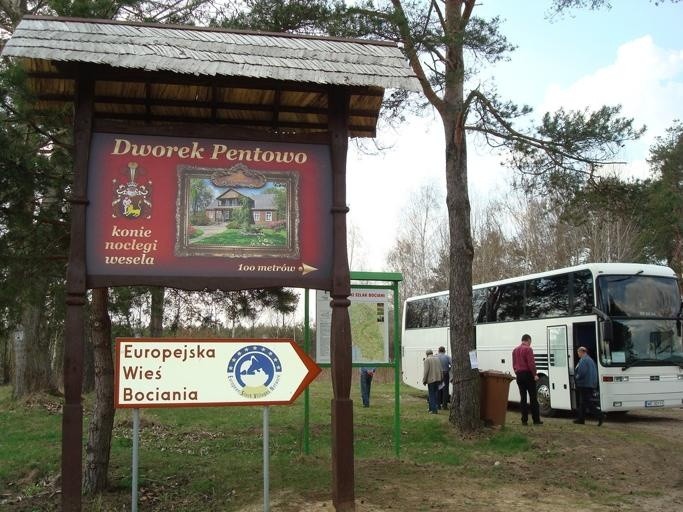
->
[401,262,683,417]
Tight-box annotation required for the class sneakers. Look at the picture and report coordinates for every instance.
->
[523,421,528,425]
[427,407,449,414]
[573,420,585,424]
[598,414,607,426]
[535,420,543,424]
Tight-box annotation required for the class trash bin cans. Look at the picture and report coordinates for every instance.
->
[479,370,516,425]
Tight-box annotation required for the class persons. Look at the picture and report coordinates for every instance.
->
[423,349,444,414]
[358,367,376,407]
[574,347,606,426]
[511,335,544,424]
[435,347,452,410]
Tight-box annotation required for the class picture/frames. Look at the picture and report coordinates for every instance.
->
[175,164,301,260]
